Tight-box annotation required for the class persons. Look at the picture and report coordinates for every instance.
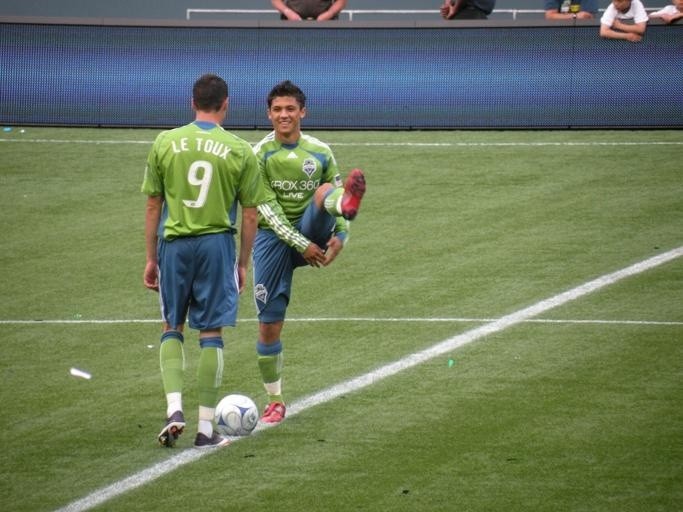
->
[598,0,650,42]
[543,0,599,22]
[271,0,349,22]
[439,0,497,20]
[141,73,267,450]
[648,0,683,24]
[252,80,366,423]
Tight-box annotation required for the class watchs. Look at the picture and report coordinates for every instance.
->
[573,13,576,20]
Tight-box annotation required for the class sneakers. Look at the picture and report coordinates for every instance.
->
[194,430,226,448]
[260,402,286,423]
[157,411,185,447]
[340,169,365,221]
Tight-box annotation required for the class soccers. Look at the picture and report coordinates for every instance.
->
[215,394,259,435]
